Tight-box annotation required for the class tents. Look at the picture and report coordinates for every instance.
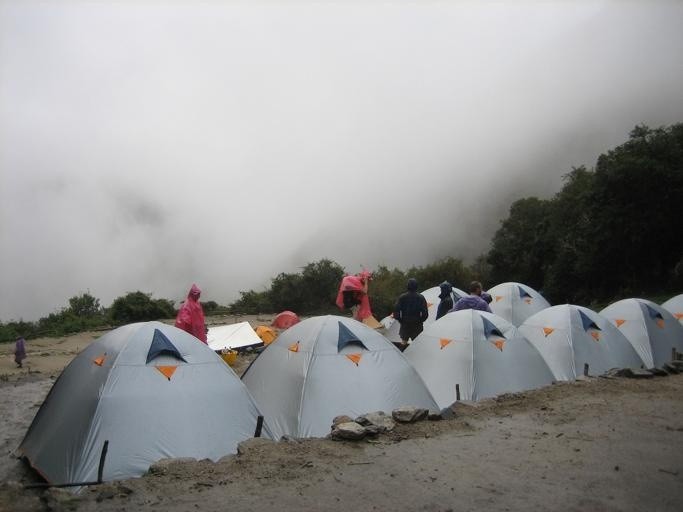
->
[381,281,682,412]
[10,318,282,490]
[239,313,443,441]
[270,310,302,329]
[251,325,277,345]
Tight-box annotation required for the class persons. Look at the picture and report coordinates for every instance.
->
[436,275,494,320]
[331,268,377,324]
[175,282,212,347]
[13,334,27,368]
[391,275,430,345]
